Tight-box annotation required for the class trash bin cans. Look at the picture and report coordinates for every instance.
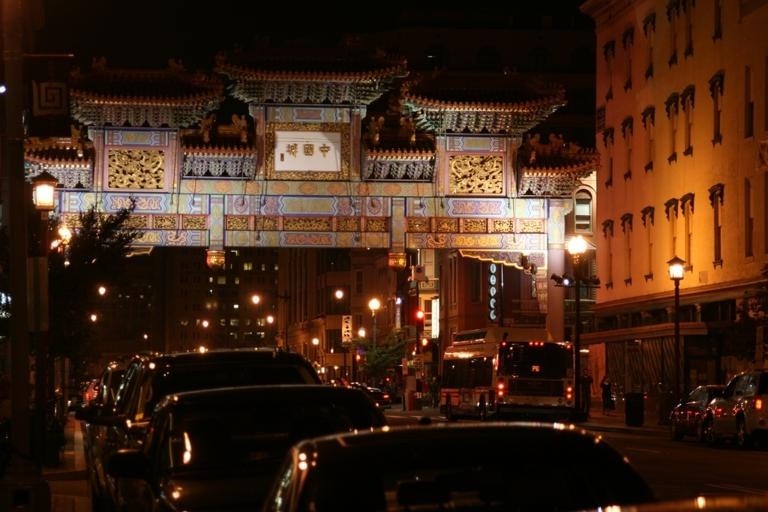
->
[624,392,644,427]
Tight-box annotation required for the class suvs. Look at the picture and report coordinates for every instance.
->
[69,346,324,512]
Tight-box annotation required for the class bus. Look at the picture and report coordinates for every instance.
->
[438,337,577,422]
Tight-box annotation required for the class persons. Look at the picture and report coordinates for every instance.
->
[579,368,595,417]
[599,375,614,416]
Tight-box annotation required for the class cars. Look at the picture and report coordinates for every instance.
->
[115,384,391,512]
[265,423,659,512]
[668,369,768,448]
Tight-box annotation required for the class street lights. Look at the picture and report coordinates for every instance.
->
[333,289,347,382]
[250,290,291,352]
[550,233,600,422]
[667,256,687,403]
